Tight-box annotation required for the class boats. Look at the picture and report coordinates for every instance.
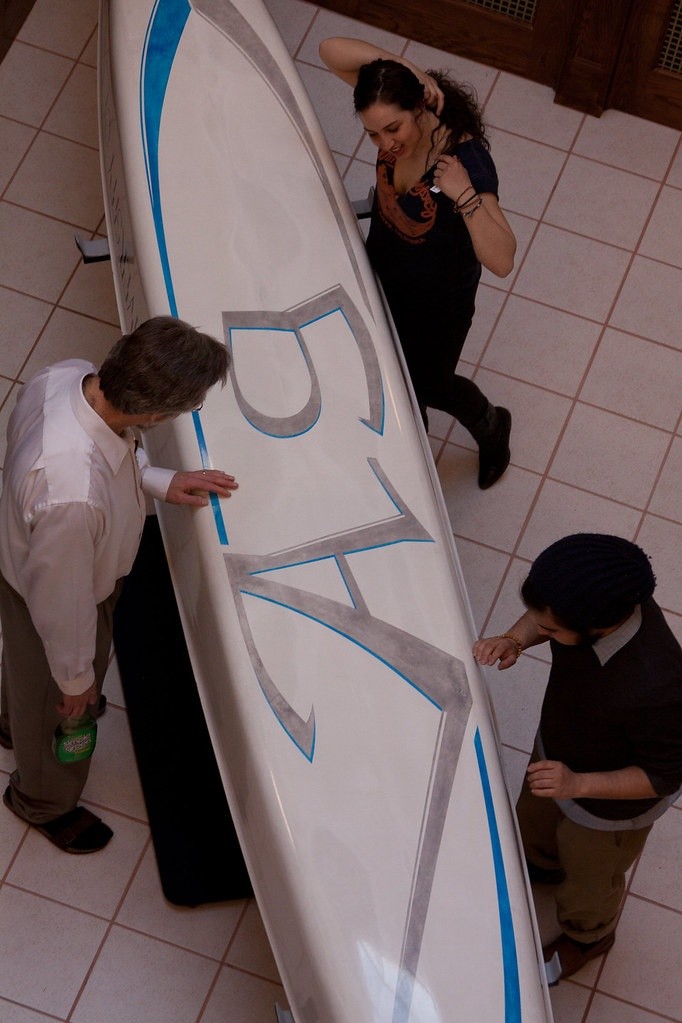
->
[93,0,554,1023]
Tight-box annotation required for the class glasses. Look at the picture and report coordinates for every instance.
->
[191,402,203,412]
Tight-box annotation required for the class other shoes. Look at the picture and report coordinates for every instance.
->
[525,859,566,883]
[542,930,615,979]
[0,694,106,749]
[478,406,512,489]
[3,784,114,854]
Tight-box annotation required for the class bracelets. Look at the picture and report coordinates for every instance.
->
[454,193,478,212]
[453,184,475,207]
[458,196,480,211]
[498,634,521,659]
[461,198,482,217]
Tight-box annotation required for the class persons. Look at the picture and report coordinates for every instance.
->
[1,313,241,856]
[315,38,518,490]
[472,533,682,980]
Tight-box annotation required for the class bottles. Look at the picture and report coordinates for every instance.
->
[52,714,98,767]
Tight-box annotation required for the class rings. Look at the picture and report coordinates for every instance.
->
[202,471,206,475]
